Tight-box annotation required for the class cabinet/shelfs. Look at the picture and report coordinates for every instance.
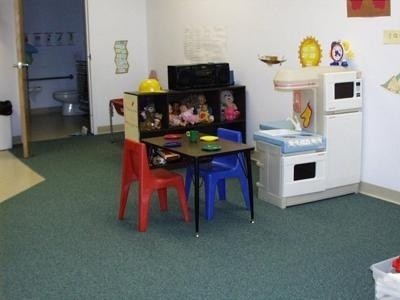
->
[122,85,247,172]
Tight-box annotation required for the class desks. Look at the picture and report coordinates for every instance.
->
[142,131,255,238]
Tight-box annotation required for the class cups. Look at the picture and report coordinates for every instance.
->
[186,131,199,143]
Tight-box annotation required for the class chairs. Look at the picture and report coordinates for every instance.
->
[118,127,251,232]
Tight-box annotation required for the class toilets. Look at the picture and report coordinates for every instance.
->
[54,90,88,116]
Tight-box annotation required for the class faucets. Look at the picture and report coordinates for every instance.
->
[286,116,302,130]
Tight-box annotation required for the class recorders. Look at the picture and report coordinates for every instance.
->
[168,63,230,90]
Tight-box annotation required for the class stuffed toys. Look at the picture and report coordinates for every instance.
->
[138,89,241,132]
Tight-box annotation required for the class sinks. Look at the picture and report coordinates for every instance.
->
[260,129,298,137]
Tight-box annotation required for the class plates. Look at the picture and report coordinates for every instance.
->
[163,141,182,148]
[201,144,222,151]
[164,134,183,140]
[200,135,218,142]
